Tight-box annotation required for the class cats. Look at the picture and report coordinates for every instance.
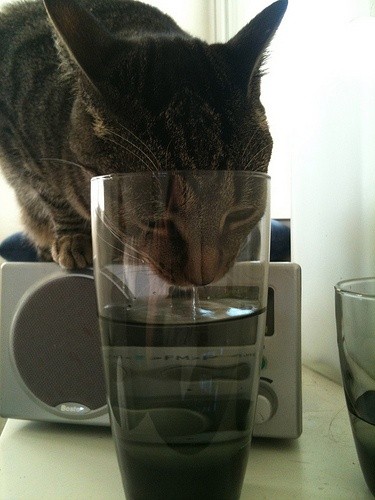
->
[1,0,288,300]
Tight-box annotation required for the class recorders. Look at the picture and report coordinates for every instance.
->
[0,256,305,443]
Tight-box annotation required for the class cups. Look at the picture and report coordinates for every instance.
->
[335,278,375,495]
[88,169,271,500]
